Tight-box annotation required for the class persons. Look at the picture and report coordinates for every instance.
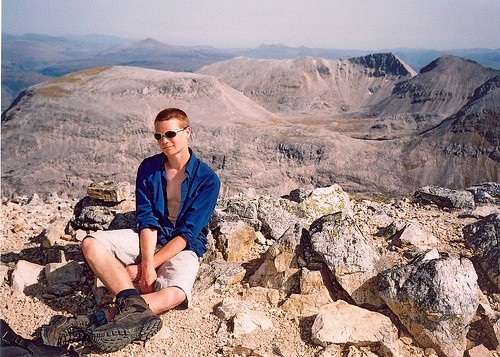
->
[39,108,221,353]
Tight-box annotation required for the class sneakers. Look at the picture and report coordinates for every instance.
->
[41,313,97,347]
[89,295,163,353]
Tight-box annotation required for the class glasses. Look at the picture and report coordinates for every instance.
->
[154,128,184,141]
